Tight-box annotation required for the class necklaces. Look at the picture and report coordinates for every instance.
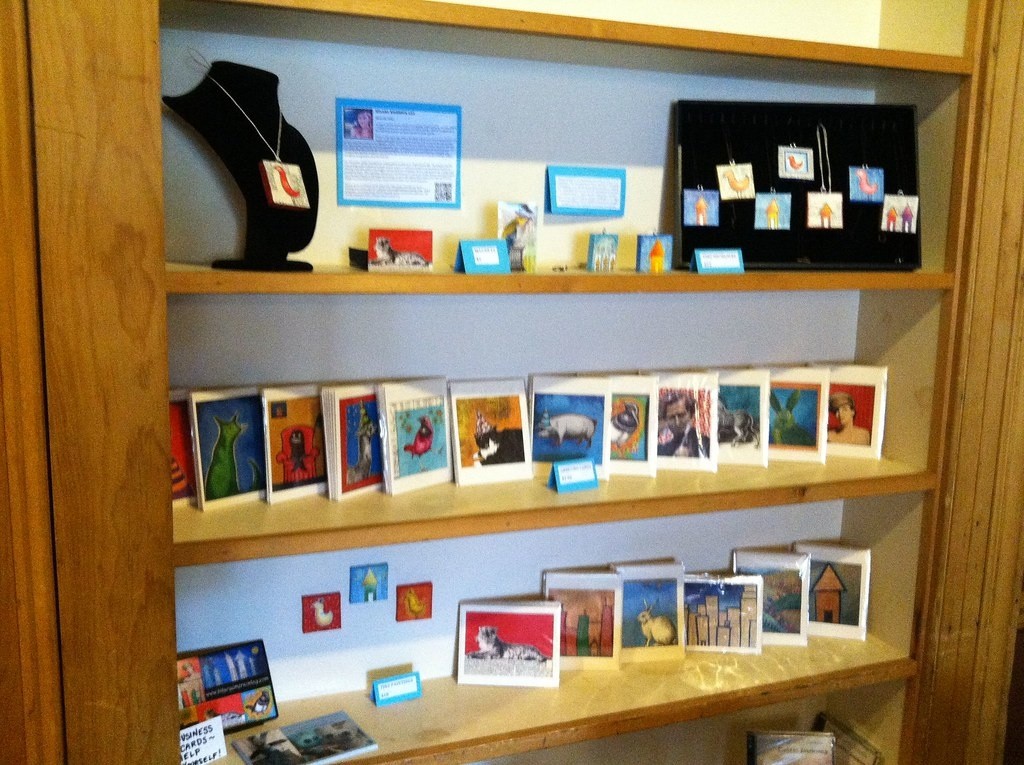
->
[185,45,310,208]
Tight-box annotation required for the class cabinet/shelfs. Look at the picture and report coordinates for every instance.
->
[0,0,1024,764]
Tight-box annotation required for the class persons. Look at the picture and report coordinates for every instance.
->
[343,108,373,140]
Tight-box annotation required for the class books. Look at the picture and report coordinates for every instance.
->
[458,541,871,688]
[169,363,889,513]
[231,710,378,764]
